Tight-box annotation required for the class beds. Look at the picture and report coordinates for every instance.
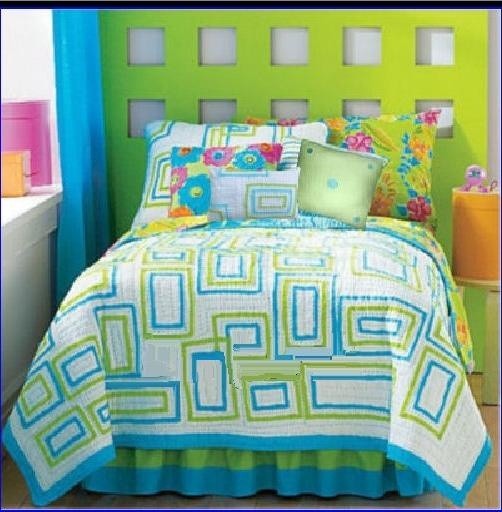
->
[58,205,440,496]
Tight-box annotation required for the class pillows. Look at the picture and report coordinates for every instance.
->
[242,106,443,229]
[297,138,387,232]
[131,120,328,232]
[206,166,300,223]
[169,143,283,218]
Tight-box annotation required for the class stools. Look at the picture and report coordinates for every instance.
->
[453,273,502,407]
